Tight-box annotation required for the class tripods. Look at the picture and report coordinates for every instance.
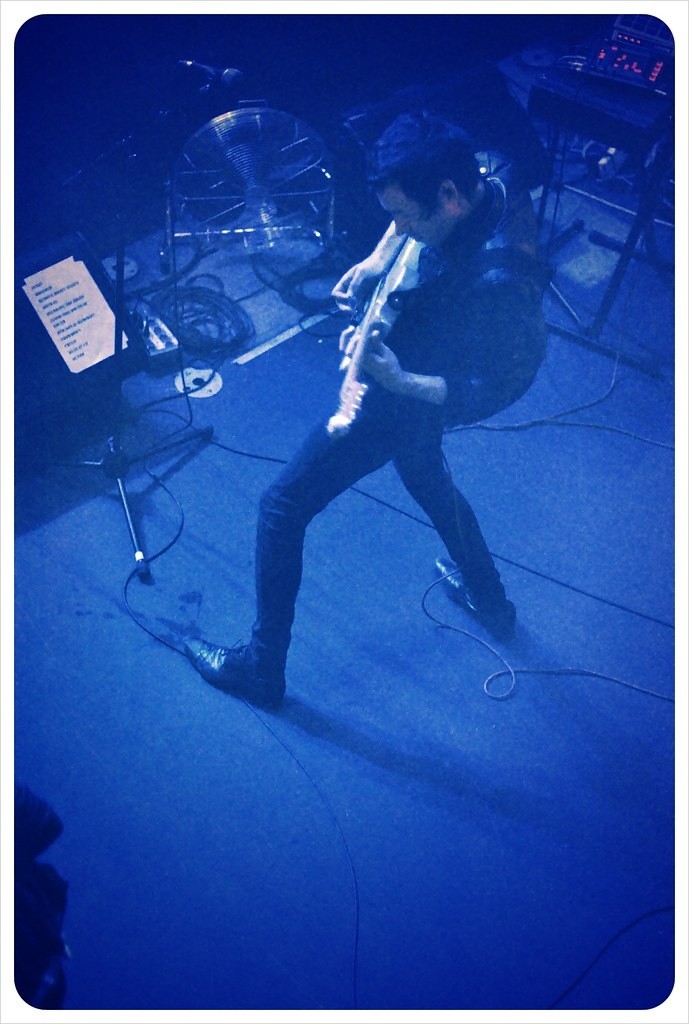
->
[15,75,217,584]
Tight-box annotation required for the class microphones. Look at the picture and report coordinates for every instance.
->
[177,59,243,90]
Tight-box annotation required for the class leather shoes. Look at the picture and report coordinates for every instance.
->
[180,635,286,706]
[434,557,516,632]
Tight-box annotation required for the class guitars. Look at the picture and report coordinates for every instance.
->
[324,237,427,437]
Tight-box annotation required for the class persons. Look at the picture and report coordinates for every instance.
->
[188,116,547,712]
